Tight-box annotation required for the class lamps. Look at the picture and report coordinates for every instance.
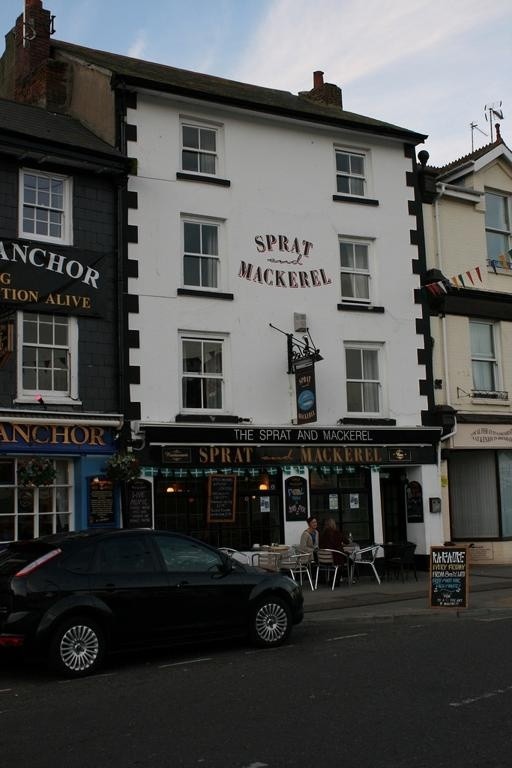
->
[257,467,270,490]
[35,394,48,410]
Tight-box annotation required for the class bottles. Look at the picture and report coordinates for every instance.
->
[349,531,353,542]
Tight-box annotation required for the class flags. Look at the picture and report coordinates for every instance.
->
[345,466,356,473]
[175,468,188,477]
[161,468,173,477]
[143,466,158,477]
[220,468,232,476]
[248,468,259,477]
[333,466,343,475]
[233,468,245,476]
[372,464,380,473]
[318,466,331,476]
[266,467,278,475]
[282,466,291,475]
[205,469,217,477]
[189,469,203,478]
[308,464,318,472]
[293,466,304,475]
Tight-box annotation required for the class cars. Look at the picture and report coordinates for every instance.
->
[1,525,304,678]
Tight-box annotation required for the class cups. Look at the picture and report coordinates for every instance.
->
[253,543,260,548]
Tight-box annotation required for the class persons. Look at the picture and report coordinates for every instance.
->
[318,515,360,584]
[301,515,325,583]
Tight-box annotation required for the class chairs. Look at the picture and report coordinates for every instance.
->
[217,542,418,592]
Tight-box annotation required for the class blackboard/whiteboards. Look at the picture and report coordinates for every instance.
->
[429,546,469,610]
[207,474,236,523]
[87,475,116,529]
[285,476,308,521]
[406,481,424,523]
[126,478,152,528]
